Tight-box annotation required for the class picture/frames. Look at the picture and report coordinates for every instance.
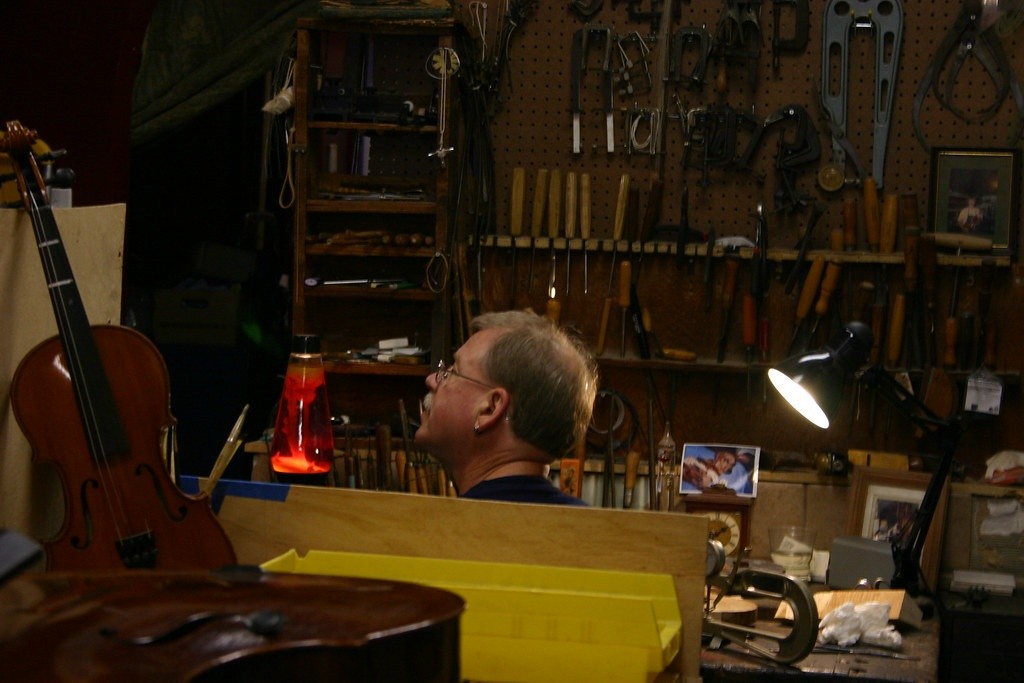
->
[927,146,1023,256]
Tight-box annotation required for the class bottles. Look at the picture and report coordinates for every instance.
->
[268,334,337,485]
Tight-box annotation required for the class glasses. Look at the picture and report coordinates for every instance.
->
[435,358,509,421]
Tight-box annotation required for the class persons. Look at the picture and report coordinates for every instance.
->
[412,308,599,508]
[956,196,983,233]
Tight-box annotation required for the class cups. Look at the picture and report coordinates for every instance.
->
[768,524,817,583]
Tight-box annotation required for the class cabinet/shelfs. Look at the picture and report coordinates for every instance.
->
[289,11,457,451]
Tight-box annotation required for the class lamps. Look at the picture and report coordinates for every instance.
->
[766,322,977,635]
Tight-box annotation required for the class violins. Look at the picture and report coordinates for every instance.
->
[0,119,235,571]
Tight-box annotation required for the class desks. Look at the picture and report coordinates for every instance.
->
[0,476,712,683]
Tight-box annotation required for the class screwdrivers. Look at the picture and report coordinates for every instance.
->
[706,175,918,406]
[506,166,632,359]
